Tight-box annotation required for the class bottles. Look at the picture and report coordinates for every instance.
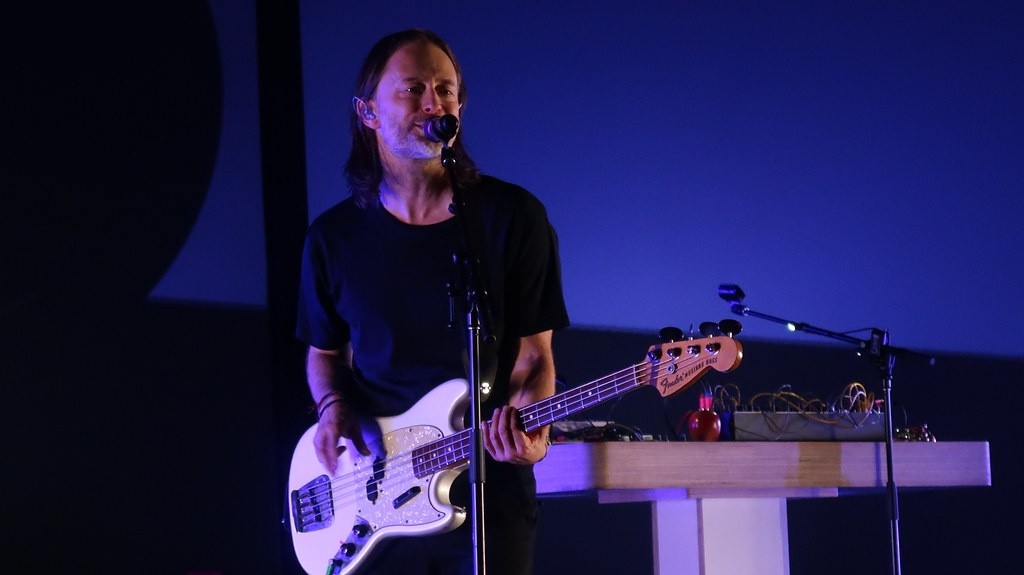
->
[688,395,721,442]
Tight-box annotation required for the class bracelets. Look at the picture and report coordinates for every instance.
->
[316,399,345,423]
[306,387,341,410]
[534,435,553,463]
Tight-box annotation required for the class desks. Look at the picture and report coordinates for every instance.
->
[533,440,991,575]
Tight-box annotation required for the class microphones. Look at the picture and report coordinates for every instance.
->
[424,113,458,142]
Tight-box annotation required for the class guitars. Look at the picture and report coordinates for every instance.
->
[286,316,746,575]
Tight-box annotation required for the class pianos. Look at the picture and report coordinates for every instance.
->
[533,440,993,499]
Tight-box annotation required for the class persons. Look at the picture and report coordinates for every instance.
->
[293,27,569,575]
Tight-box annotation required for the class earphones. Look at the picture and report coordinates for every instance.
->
[363,107,376,119]
